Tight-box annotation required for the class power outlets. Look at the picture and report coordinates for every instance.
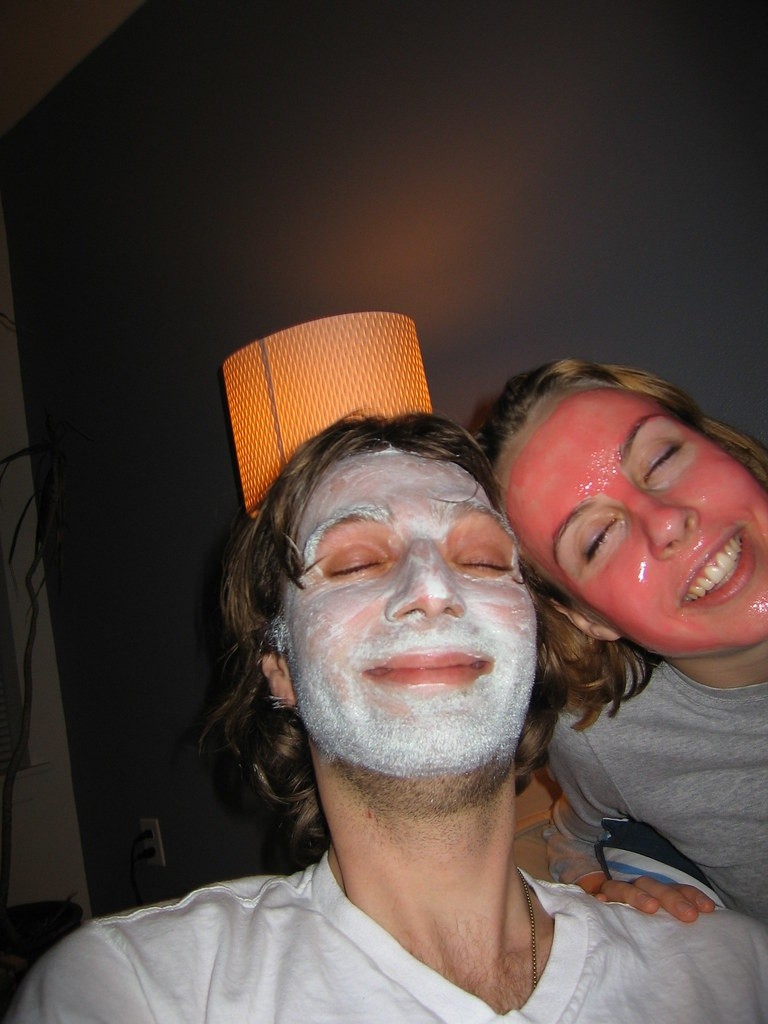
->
[139,819,166,867]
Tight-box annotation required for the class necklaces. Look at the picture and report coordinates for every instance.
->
[515,868,538,988]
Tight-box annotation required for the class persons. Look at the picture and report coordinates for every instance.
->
[7,407,767,1023]
[470,355,768,930]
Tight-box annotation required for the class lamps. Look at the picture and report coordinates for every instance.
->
[222,310,434,519]
